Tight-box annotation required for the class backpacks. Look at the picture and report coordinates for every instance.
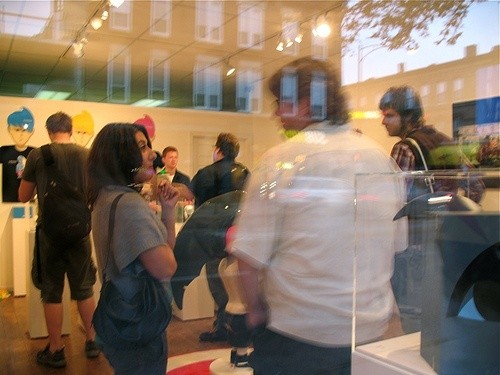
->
[41,144,91,246]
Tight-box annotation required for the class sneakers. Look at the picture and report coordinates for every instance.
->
[86,340,100,358]
[36,343,67,367]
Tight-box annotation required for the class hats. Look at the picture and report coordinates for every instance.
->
[226,225,238,253]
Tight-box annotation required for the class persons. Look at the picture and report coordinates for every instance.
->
[231,55,407,375]
[139,145,191,199]
[218,225,265,368]
[18,114,101,366]
[380,85,486,333]
[86,123,178,375]
[174,133,251,342]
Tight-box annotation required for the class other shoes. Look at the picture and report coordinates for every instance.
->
[199,327,227,343]
[235,353,250,367]
[231,350,238,364]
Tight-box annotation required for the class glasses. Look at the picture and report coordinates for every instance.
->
[276,98,286,106]
[212,145,218,151]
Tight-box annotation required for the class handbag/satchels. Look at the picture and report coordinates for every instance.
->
[92,192,172,351]
